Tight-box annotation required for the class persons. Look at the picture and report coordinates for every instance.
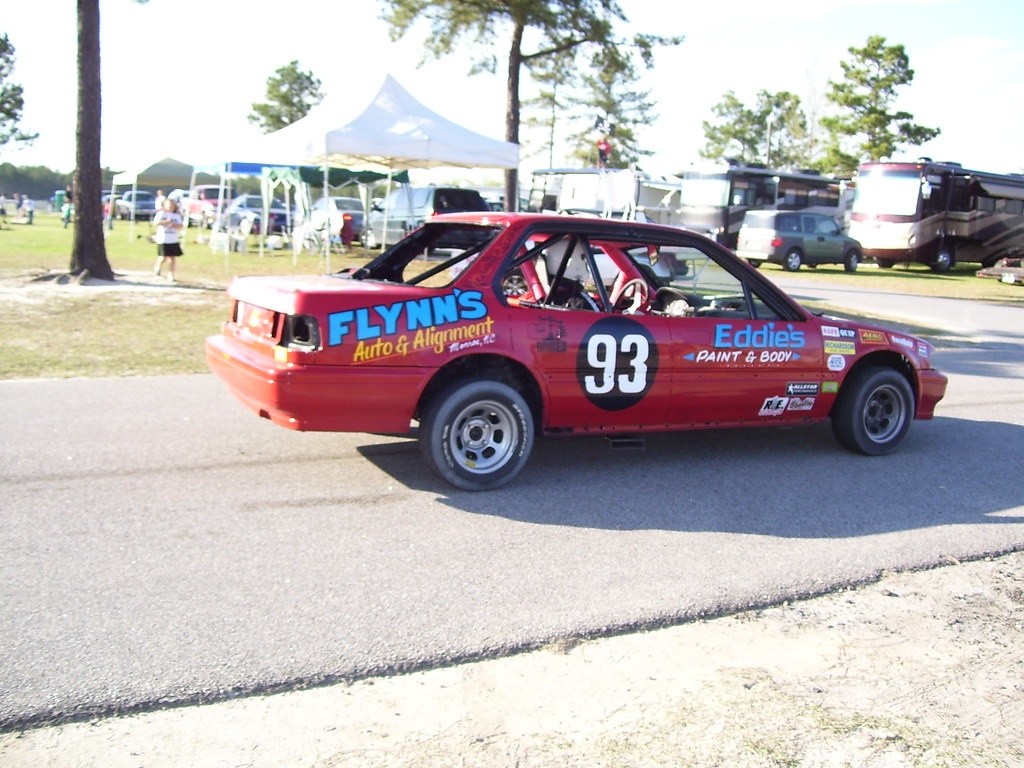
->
[153,185,184,282]
[597,137,611,170]
[1,192,35,224]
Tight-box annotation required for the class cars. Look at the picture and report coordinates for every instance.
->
[204,212,948,493]
[220,195,296,234]
[301,198,367,243]
[166,188,190,214]
[186,185,240,224]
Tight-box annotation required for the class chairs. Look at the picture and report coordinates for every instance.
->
[231,217,255,253]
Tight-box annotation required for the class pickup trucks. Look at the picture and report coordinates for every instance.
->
[115,191,157,221]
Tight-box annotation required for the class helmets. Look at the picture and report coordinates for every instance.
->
[547,238,589,282]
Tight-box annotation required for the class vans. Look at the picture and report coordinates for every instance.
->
[736,210,863,272]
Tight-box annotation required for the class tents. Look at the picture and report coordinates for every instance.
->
[212,64,524,275]
[109,154,238,243]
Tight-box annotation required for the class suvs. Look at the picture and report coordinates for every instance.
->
[364,185,491,248]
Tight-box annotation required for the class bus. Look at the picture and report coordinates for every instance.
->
[528,168,682,224]
[850,157,1024,273]
[680,164,855,254]
